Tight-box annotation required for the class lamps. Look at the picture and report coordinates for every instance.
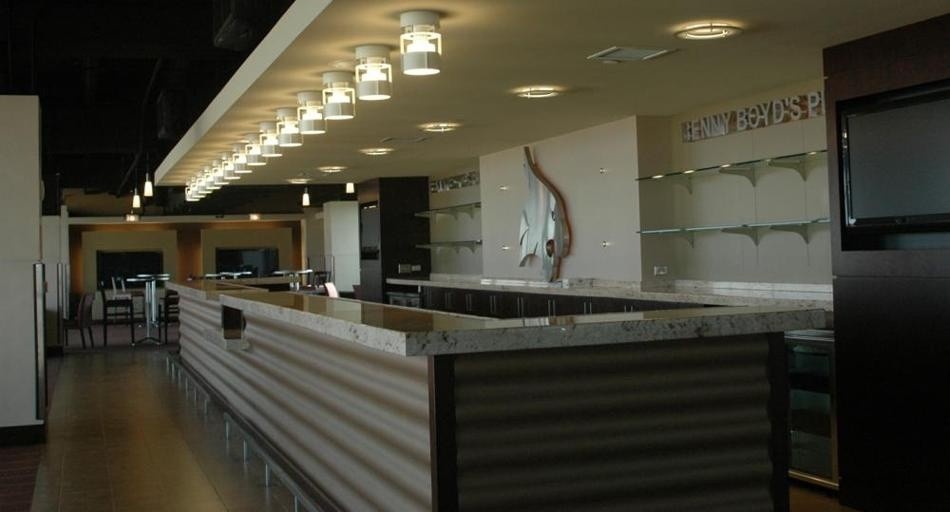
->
[399,12,443,75]
[297,91,326,133]
[346,177,354,194]
[321,70,358,122]
[185,109,299,206]
[125,144,155,223]
[353,46,393,100]
[301,188,310,205]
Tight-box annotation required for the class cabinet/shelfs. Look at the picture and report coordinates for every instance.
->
[386,285,705,319]
[413,202,482,256]
[634,149,830,248]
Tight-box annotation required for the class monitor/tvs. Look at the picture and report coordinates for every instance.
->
[97,250,164,289]
[838,88,950,230]
[215,246,279,278]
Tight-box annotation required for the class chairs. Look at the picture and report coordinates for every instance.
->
[313,270,331,290]
[157,286,180,346]
[98,276,145,348]
[63,292,96,350]
